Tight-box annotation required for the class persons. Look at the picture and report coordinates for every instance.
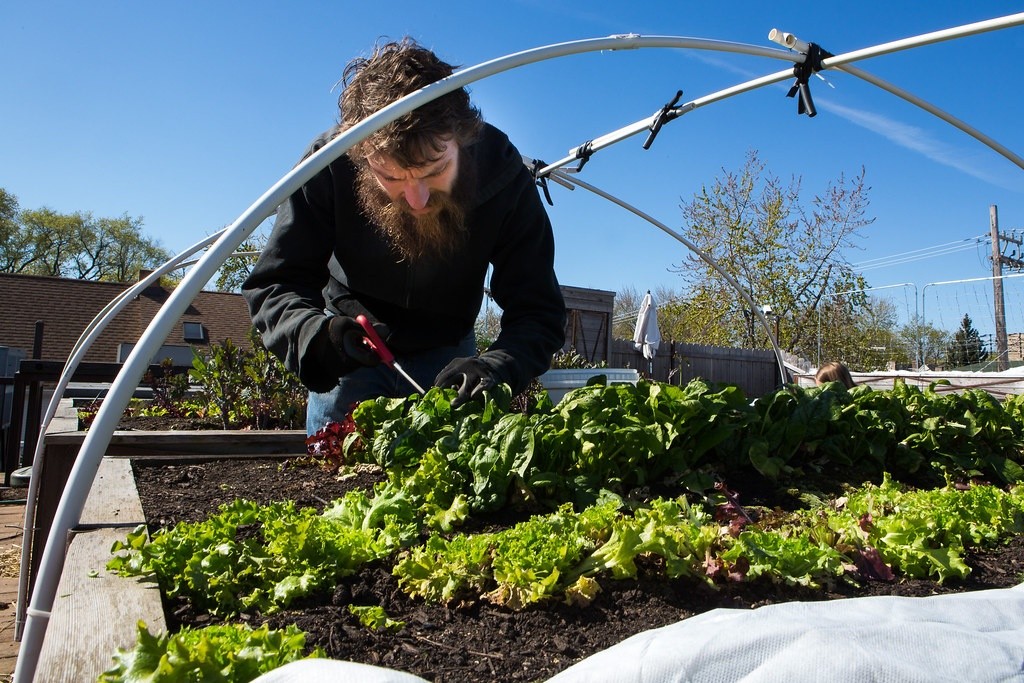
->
[815,361,859,389]
[242,31,568,455]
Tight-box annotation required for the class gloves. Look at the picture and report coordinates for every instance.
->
[310,316,395,376]
[433,348,529,407]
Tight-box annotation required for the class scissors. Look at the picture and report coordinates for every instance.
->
[357,314,427,395]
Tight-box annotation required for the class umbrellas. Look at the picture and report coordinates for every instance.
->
[633,289,661,378]
